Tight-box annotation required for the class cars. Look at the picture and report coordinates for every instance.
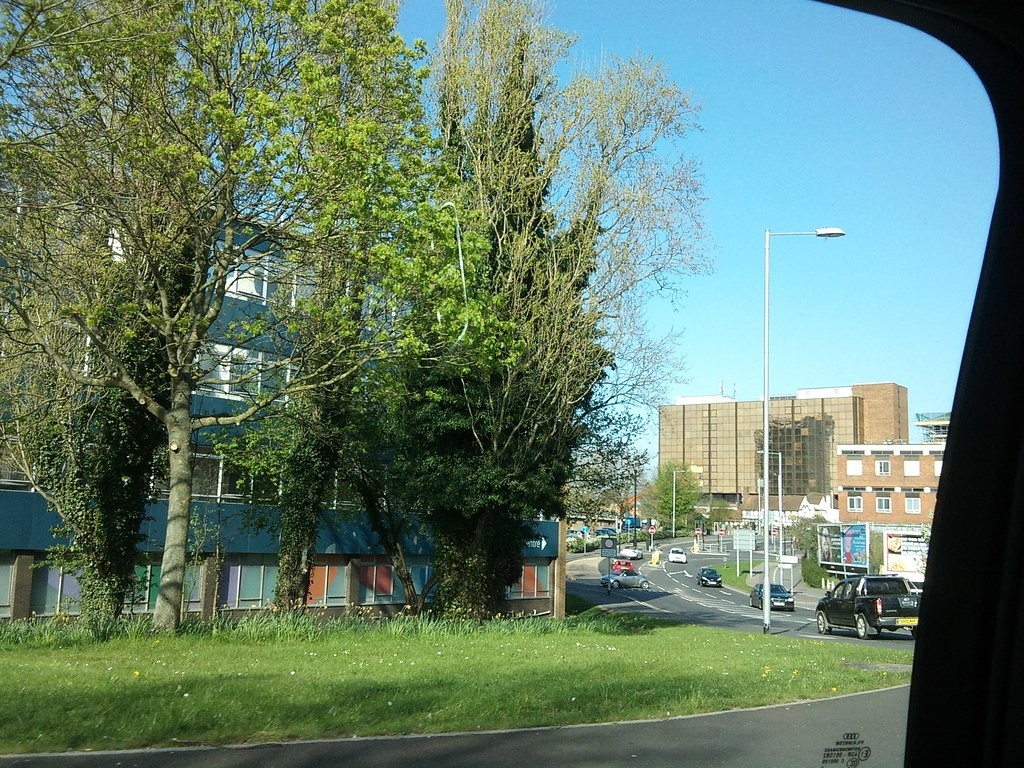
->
[620,545,645,559]
[748,584,796,609]
[697,568,722,588]
[567,530,592,540]
[601,569,652,590]
[612,560,634,571]
[594,528,619,537]
[668,548,689,563]
[612,555,633,566]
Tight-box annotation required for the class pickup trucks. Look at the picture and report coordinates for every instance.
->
[814,573,922,640]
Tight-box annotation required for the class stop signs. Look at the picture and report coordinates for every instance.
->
[719,529,725,535]
[771,530,778,536]
[648,526,656,534]
[695,528,702,535]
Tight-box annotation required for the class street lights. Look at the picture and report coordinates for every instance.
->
[762,226,863,627]
[756,450,785,586]
[633,469,638,547]
[673,470,686,537]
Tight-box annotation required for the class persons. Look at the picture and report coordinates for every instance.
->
[822,529,831,562]
